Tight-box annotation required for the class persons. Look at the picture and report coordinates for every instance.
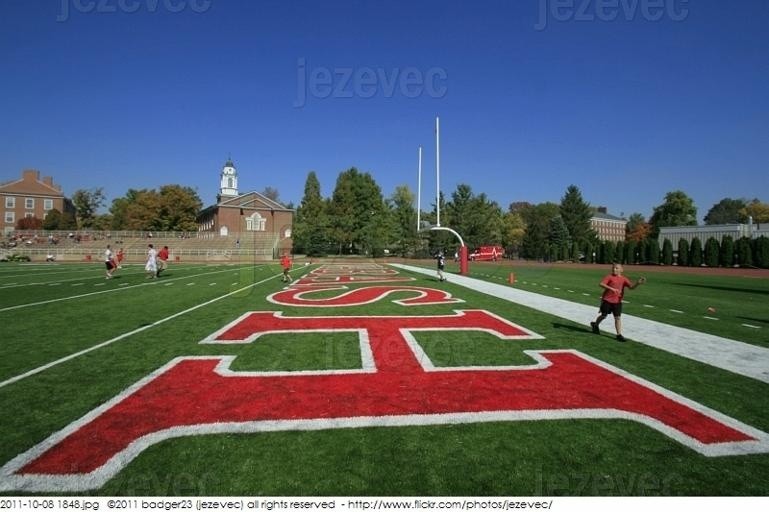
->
[103,230,169,280]
[434,248,446,281]
[276,250,293,283]
[590,261,646,342]
[0,229,103,262]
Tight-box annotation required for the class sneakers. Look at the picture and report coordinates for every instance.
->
[590,321,600,335]
[616,334,627,342]
